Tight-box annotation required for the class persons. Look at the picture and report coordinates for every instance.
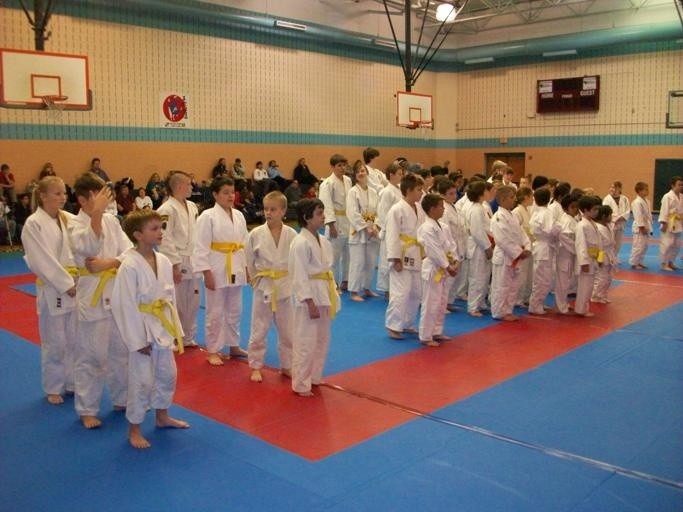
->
[546,177,558,196]
[15,192,33,241]
[574,197,600,318]
[553,179,571,203]
[628,181,654,270]
[658,176,683,272]
[268,160,289,189]
[450,170,462,189]
[231,158,248,183]
[556,194,579,316]
[490,170,503,192]
[392,155,408,172]
[252,161,279,189]
[489,185,532,322]
[284,178,309,208]
[146,173,160,197]
[286,198,342,398]
[304,182,320,200]
[242,191,298,382]
[590,205,616,304]
[437,177,462,314]
[156,169,200,349]
[603,180,631,267]
[135,187,154,210]
[462,180,494,318]
[116,184,135,216]
[470,171,487,184]
[482,161,505,191]
[519,174,532,188]
[20,173,78,406]
[503,165,517,192]
[345,159,380,302]
[384,170,426,341]
[361,146,385,189]
[430,160,450,179]
[572,184,596,201]
[416,193,460,347]
[317,153,352,295]
[191,177,249,367]
[39,162,56,179]
[410,162,422,174]
[429,175,448,195]
[475,172,487,180]
[527,186,562,316]
[211,158,228,177]
[293,157,318,191]
[533,174,549,191]
[90,158,114,192]
[65,168,137,432]
[1,164,17,203]
[417,168,433,200]
[108,208,191,451]
[372,160,404,305]
